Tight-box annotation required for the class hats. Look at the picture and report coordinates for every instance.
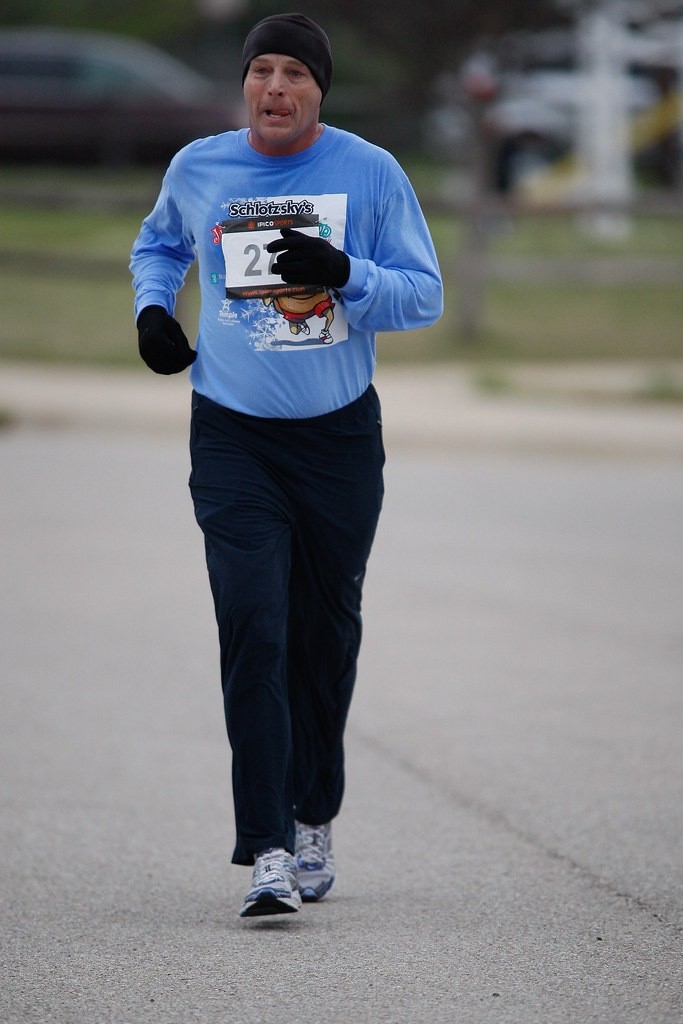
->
[242,12,334,106]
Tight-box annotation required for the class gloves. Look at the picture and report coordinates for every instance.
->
[267,226,351,288]
[137,305,198,375]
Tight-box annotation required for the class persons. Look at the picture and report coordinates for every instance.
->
[464,37,566,205]
[129,12,444,914]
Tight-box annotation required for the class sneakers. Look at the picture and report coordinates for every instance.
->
[294,820,336,900]
[239,847,303,918]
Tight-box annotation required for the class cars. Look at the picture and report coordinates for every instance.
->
[0,26,248,170]
